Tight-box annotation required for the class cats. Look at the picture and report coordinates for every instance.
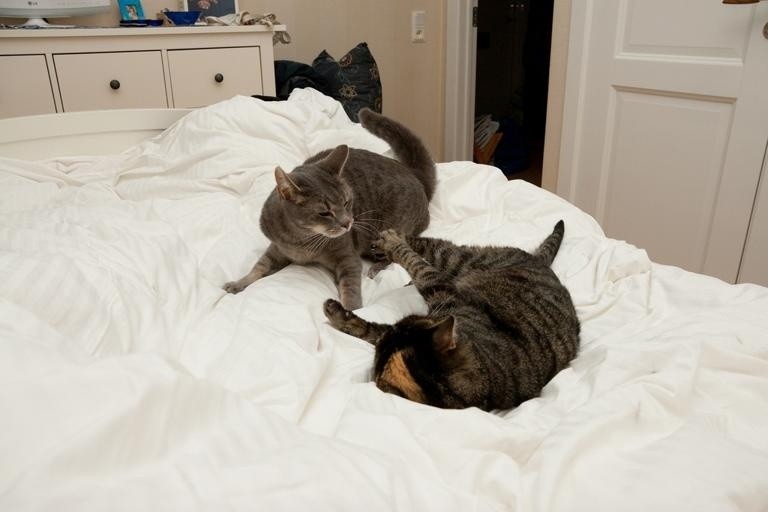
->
[323,219,581,411]
[223,107,440,314]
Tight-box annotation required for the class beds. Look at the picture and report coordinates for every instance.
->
[0,86,768,512]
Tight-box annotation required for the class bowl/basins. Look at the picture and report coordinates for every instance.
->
[163,10,204,26]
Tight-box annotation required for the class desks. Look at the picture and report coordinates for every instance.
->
[0,23,288,121]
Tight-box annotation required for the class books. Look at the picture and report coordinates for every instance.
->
[474,114,501,165]
